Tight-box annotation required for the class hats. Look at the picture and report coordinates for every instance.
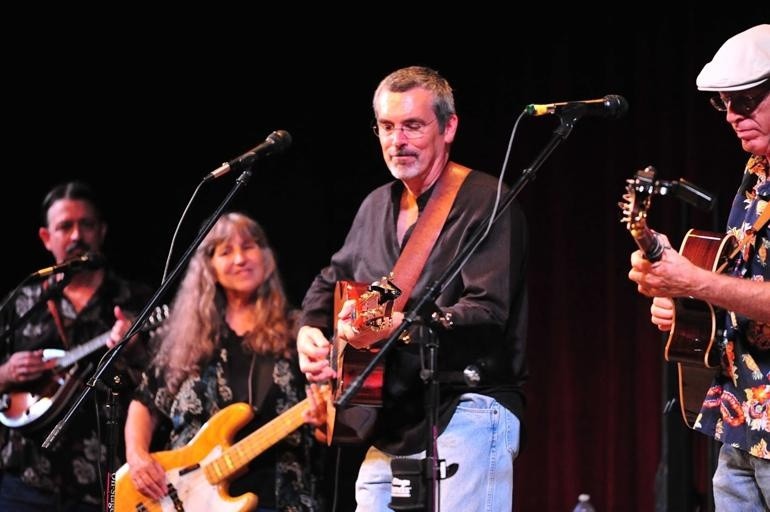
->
[696,23,770,93]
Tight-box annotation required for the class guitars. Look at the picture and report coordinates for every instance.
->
[327,277,402,447]
[618,166,739,429]
[108,385,329,512]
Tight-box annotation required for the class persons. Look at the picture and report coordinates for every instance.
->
[0,182,150,512]
[124,213,327,512]
[629,23,770,512]
[296,65,529,512]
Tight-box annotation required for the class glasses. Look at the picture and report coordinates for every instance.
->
[709,91,766,115]
[373,117,438,139]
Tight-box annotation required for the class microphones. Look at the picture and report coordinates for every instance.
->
[527,94,628,116]
[203,129,292,181]
[31,250,105,279]
